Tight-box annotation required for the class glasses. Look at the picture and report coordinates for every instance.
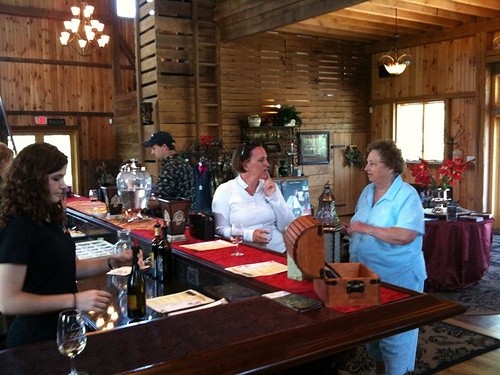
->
[240,140,258,155]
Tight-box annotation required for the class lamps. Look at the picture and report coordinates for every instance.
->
[59,1,110,57]
[378,6,413,75]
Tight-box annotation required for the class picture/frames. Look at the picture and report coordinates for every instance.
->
[299,131,330,164]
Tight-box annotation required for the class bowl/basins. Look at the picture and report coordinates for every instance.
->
[88,207,108,218]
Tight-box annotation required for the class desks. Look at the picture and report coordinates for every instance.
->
[0,194,467,374]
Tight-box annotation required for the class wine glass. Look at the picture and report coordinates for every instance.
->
[261,226,273,243]
[230,222,244,257]
[55,309,88,374]
[88,189,100,209]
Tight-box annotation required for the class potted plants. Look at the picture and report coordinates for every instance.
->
[270,102,303,128]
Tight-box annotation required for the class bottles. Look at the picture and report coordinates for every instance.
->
[114,229,135,258]
[155,223,172,283]
[126,244,147,318]
[150,224,162,279]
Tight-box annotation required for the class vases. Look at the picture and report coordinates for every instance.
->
[100,187,122,215]
[432,191,444,213]
[152,151,197,235]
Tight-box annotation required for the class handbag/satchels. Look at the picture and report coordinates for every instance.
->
[190,211,215,240]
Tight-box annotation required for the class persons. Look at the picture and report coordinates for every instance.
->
[0,141,144,346]
[0,142,14,182]
[143,131,202,214]
[342,139,427,375]
[212,141,296,254]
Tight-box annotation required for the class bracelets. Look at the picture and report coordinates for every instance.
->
[107,256,112,270]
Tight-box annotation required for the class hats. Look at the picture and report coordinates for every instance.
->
[142,131,175,147]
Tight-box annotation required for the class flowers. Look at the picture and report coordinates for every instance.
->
[406,156,469,190]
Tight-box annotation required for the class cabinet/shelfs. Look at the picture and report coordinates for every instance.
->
[242,127,297,179]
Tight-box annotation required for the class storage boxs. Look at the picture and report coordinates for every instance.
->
[282,214,382,310]
